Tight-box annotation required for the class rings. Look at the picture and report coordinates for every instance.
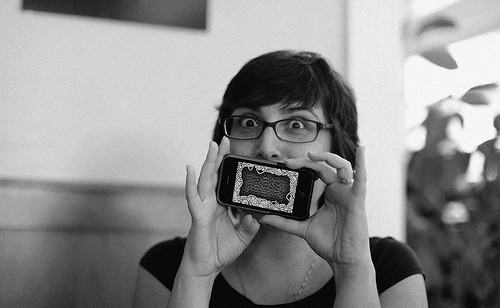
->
[339,177,355,183]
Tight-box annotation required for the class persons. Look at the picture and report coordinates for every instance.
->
[398,93,475,308]
[465,110,500,189]
[134,46,430,307]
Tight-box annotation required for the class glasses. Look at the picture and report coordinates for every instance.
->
[220,114,337,143]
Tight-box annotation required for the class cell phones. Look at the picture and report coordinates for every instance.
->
[215,154,315,221]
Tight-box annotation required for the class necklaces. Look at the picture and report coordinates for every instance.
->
[232,253,319,306]
